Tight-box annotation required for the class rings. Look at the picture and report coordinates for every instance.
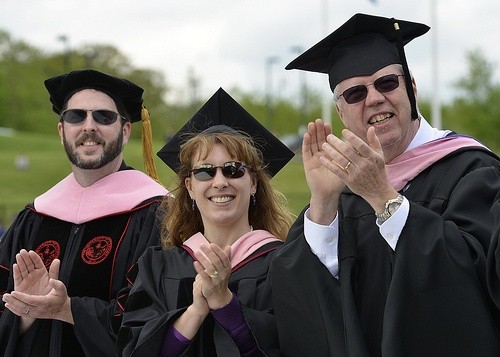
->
[25,311,29,315]
[209,270,218,278]
[344,162,351,170]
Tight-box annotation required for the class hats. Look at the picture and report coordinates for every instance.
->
[156,86,295,181]
[43,70,159,183]
[285,13,431,121]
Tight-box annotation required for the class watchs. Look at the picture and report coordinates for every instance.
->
[375,194,404,226]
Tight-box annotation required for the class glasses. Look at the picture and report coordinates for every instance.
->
[61,108,127,125]
[187,161,253,181]
[337,74,404,104]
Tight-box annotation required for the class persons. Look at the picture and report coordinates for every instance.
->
[114,86,294,357]
[0,70,178,357]
[264,11,500,357]
